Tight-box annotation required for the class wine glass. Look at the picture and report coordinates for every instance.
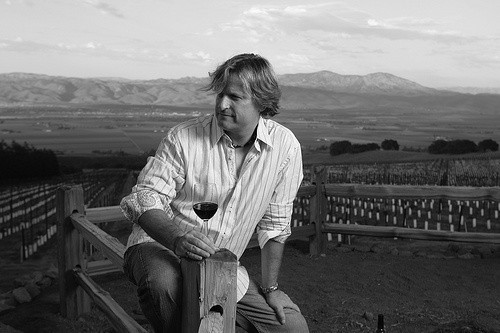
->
[192,183,218,237]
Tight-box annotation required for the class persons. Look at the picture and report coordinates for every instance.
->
[119,54,310,333]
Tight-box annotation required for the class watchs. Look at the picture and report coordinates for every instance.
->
[259,282,279,294]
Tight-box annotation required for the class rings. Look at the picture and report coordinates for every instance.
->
[185,251,189,258]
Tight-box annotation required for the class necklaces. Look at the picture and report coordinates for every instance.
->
[232,132,256,148]
